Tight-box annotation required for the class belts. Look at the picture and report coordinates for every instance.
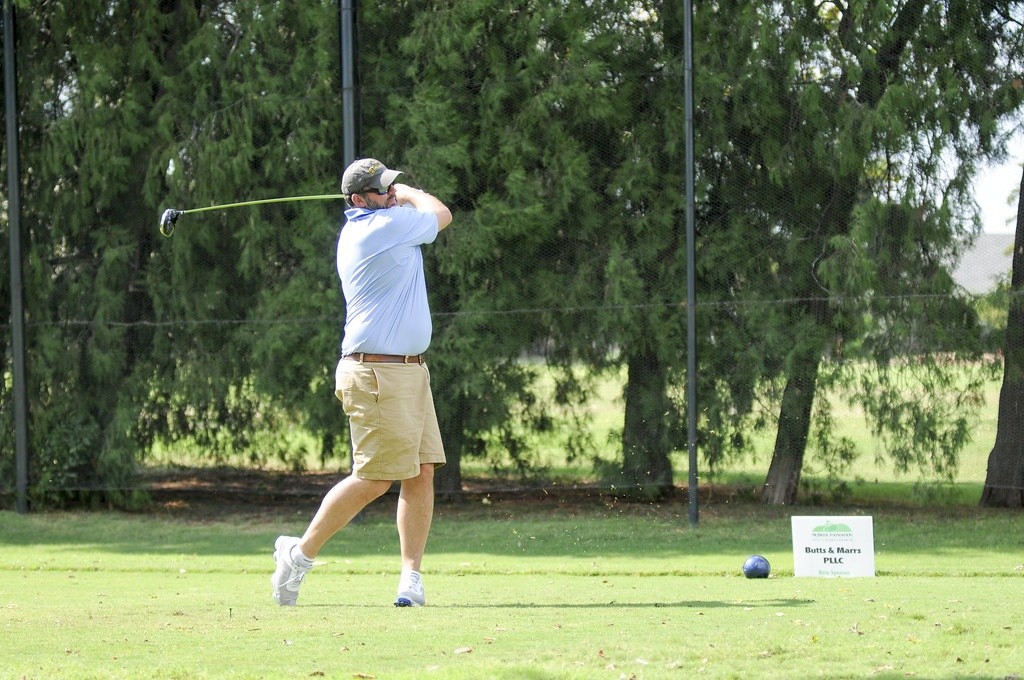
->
[344,350,425,366]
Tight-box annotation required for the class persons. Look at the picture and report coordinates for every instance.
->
[271,158,453,607]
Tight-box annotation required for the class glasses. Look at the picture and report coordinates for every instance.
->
[355,181,395,195]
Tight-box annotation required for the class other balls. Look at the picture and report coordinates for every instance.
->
[742,554,771,579]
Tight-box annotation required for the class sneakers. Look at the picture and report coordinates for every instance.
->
[271,535,313,607]
[394,569,426,607]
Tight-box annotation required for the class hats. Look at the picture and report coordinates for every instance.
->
[341,158,404,195]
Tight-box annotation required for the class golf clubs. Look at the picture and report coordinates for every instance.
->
[159,193,344,237]
